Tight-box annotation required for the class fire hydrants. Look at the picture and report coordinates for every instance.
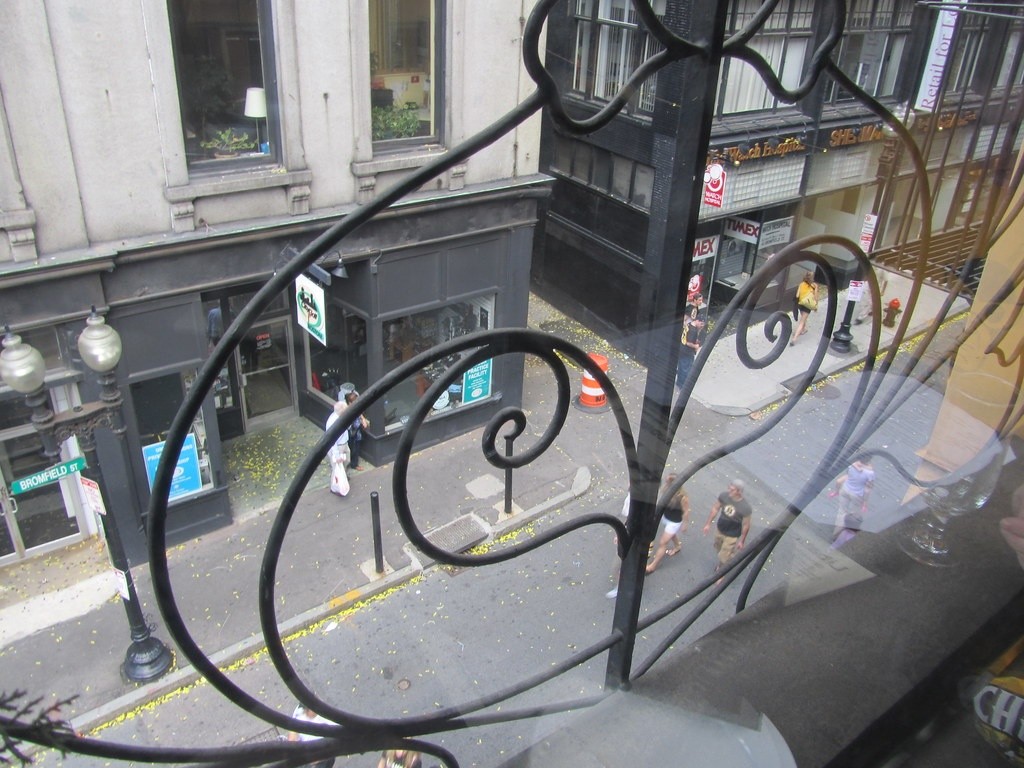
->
[882,298,902,327]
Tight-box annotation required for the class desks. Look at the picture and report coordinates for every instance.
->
[370,71,426,108]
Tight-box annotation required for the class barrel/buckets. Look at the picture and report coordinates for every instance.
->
[580,353,608,407]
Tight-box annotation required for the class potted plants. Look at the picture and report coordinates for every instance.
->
[371,104,420,140]
[200,127,255,160]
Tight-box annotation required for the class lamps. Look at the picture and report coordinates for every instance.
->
[244,87,268,154]
[320,250,349,279]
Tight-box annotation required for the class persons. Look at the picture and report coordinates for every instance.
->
[828,513,864,549]
[790,271,818,347]
[288,702,340,768]
[378,737,422,768]
[675,316,705,394]
[206,297,262,379]
[703,479,752,574]
[644,473,689,576]
[827,461,873,513]
[682,293,702,333]
[326,400,351,496]
[345,392,368,471]
[829,450,875,540]
[603,482,653,600]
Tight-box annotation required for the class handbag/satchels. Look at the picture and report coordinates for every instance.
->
[329,462,351,497]
[349,436,357,457]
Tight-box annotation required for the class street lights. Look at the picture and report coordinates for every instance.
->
[829,101,915,352]
[0,317,174,682]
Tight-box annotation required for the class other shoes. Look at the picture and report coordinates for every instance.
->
[355,465,364,471]
[828,491,839,498]
[667,546,682,556]
[861,503,867,513]
[645,565,657,575]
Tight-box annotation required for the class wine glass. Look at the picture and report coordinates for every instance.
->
[892,369,1024,570]
